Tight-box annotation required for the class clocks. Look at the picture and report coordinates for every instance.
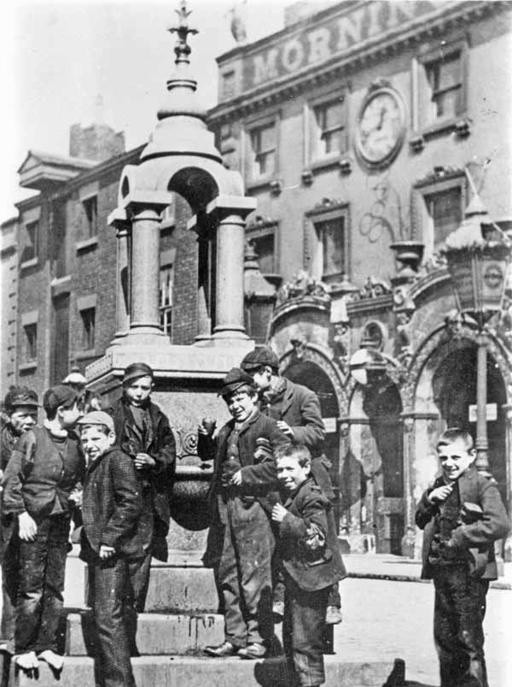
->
[352,80,410,172]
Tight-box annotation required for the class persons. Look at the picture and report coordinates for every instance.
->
[1,384,88,670]
[269,441,346,687]
[0,387,42,654]
[415,427,511,686]
[239,346,348,626]
[107,362,176,611]
[68,412,144,686]
[196,367,293,660]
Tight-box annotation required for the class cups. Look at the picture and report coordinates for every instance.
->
[459,501,483,526]
[202,416,217,435]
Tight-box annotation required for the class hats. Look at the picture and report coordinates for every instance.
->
[123,363,157,387]
[5,387,44,409]
[42,384,78,414]
[215,366,255,398]
[241,347,280,372]
[79,411,117,436]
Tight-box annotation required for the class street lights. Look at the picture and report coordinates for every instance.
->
[443,192,512,572]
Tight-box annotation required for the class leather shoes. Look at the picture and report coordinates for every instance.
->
[238,641,272,659]
[204,644,237,658]
